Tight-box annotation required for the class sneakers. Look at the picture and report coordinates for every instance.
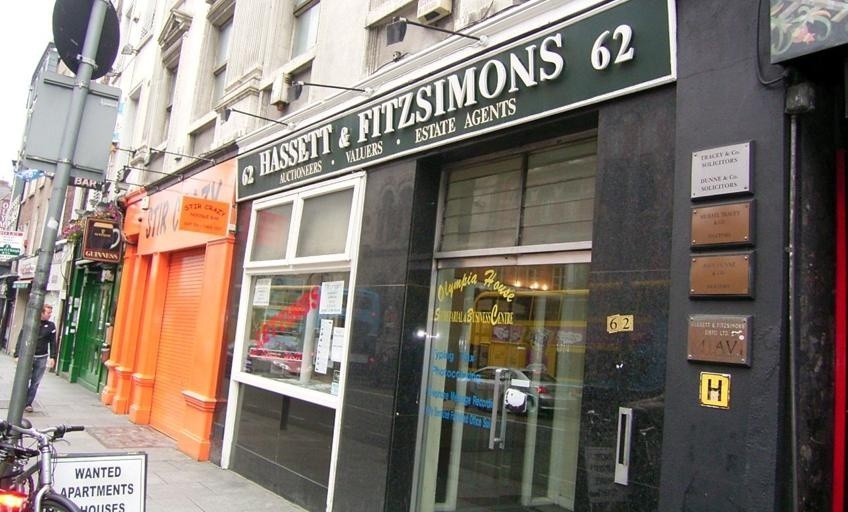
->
[25,406,33,411]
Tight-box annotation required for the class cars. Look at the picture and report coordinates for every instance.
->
[227,330,307,383]
[361,348,402,385]
[460,364,580,417]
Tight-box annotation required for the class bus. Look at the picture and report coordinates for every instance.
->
[230,280,378,380]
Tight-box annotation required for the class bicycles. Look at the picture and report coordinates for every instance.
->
[1,416,90,512]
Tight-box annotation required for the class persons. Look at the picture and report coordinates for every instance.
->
[13,303,59,413]
